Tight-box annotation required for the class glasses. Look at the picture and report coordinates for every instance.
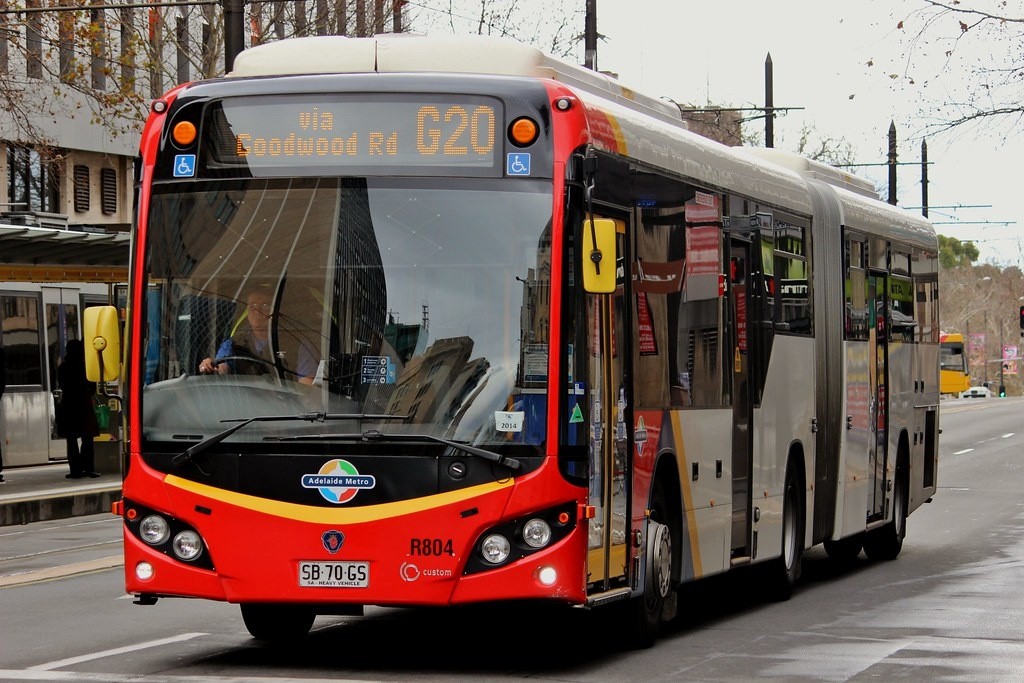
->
[248,301,272,314]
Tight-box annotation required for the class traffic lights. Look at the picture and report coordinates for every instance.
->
[982,381,989,388]
[999,386,1006,398]
[1020,306,1024,329]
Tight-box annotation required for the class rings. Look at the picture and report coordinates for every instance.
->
[201,367,205,369]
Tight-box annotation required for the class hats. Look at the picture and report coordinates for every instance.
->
[66,339,85,360]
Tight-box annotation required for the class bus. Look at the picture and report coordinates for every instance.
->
[84,31,941,641]
[958,386,991,399]
[939,334,971,395]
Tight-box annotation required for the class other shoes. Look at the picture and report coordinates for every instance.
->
[66,471,82,480]
[81,470,102,479]
[0,475,6,483]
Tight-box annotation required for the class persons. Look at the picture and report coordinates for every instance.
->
[56,340,101,477]
[199,288,318,385]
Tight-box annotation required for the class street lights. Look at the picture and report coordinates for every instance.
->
[966,275,991,365]
